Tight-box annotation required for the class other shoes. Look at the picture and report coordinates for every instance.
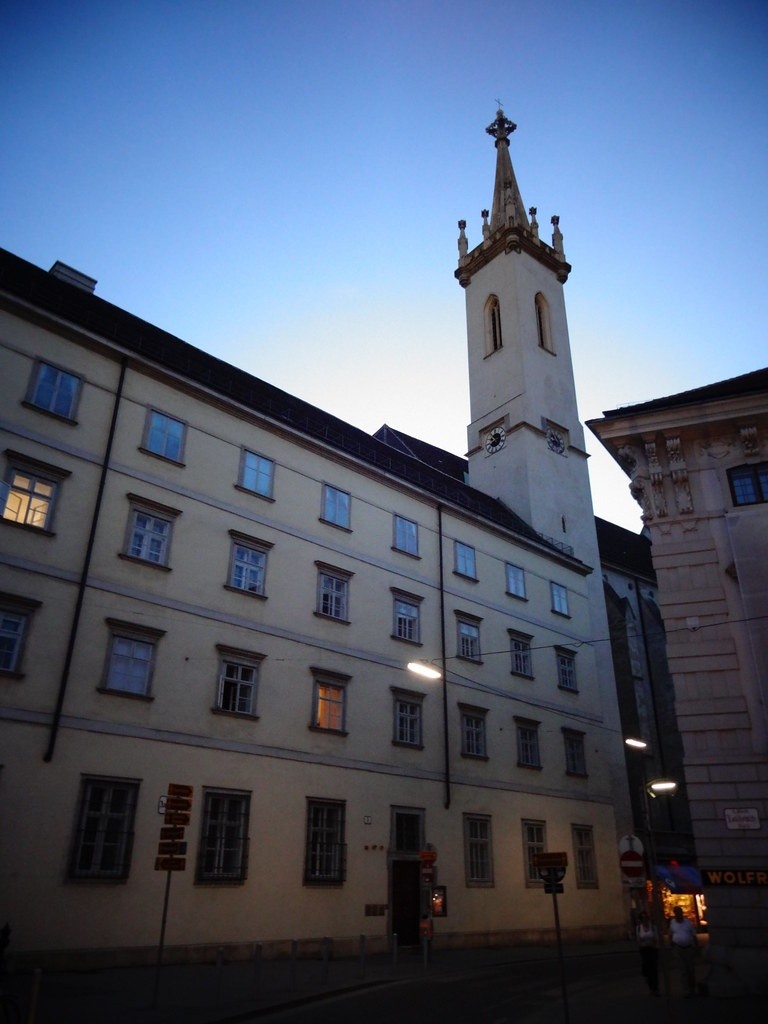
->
[650,982,659,999]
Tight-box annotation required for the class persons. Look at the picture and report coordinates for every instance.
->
[667,906,701,999]
[635,911,660,998]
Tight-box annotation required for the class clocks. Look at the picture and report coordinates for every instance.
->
[545,428,565,453]
[485,426,506,454]
